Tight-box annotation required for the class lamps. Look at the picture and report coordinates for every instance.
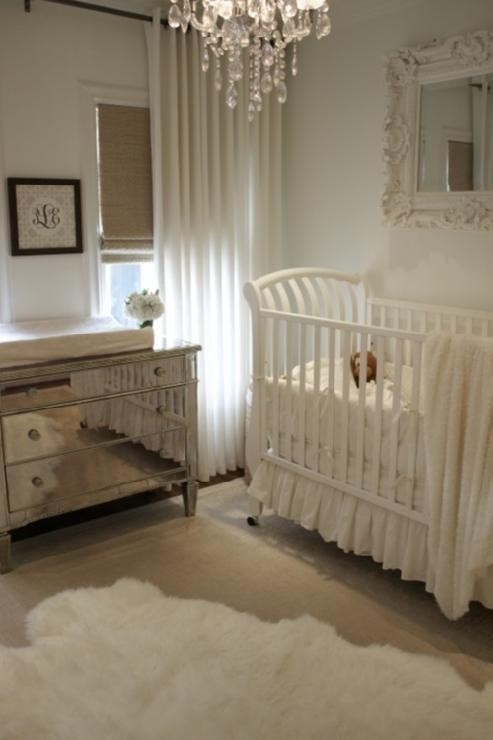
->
[166,0,331,124]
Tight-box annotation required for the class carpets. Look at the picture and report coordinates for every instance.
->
[0,575,492,740]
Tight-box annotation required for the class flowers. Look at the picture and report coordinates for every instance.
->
[125,290,165,329]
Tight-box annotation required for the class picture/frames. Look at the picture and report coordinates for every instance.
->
[6,177,82,258]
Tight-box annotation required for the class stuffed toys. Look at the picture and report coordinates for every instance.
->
[349,350,382,388]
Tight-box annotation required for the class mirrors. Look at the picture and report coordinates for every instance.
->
[383,30,492,231]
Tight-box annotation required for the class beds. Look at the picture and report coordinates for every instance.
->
[243,267,491,614]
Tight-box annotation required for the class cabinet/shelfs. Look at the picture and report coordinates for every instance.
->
[0,352,202,572]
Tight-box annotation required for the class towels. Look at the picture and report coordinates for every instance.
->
[423,333,493,624]
